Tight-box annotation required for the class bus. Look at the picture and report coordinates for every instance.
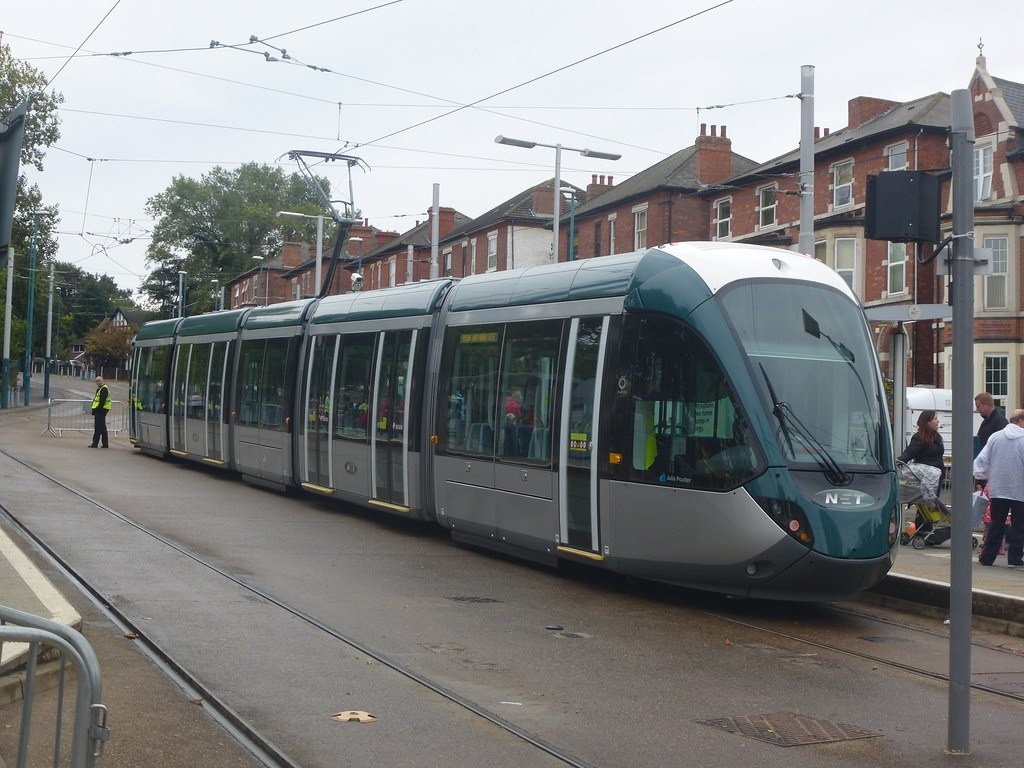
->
[129,150,900,606]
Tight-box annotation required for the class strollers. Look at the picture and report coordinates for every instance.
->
[895,459,978,550]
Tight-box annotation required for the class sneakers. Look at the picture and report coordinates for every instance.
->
[1008,558,1024,567]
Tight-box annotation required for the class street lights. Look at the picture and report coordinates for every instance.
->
[210,279,219,313]
[24,209,52,406]
[178,271,187,318]
[560,187,575,262]
[275,210,364,299]
[250,253,270,306]
[493,133,623,266]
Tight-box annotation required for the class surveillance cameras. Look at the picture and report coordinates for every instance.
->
[351,273,363,284]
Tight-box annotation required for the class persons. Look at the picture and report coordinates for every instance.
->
[88,376,112,448]
[896,407,946,500]
[319,389,403,441]
[973,392,1024,566]
[130,380,143,436]
[492,388,538,456]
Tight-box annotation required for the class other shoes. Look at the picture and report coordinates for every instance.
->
[101,446,108,448]
[978,542,1005,555]
[88,445,97,448]
